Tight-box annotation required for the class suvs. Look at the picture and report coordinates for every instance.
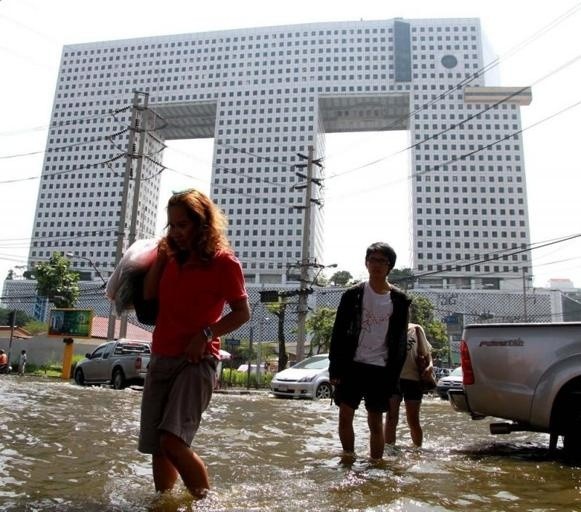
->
[436,367,466,400]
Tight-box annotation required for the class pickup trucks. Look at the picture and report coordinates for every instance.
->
[448,321,581,463]
[75,338,153,390]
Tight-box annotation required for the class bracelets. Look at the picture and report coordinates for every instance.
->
[203,327,213,342]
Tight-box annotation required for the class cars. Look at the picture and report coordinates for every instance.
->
[236,361,297,378]
[432,368,453,377]
[269,351,335,403]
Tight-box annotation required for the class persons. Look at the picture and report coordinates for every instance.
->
[18,349,27,376]
[0,348,7,370]
[329,241,411,459]
[384,310,433,448]
[135,191,251,499]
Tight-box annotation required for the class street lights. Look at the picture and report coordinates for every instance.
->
[296,264,338,362]
[66,252,116,340]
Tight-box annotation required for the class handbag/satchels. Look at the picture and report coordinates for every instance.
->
[417,355,436,390]
[128,268,157,326]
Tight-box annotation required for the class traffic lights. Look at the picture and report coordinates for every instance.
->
[6,311,14,326]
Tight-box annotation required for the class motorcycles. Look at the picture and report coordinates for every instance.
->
[0,365,13,374]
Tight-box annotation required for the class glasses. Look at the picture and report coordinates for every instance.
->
[367,256,389,267]
